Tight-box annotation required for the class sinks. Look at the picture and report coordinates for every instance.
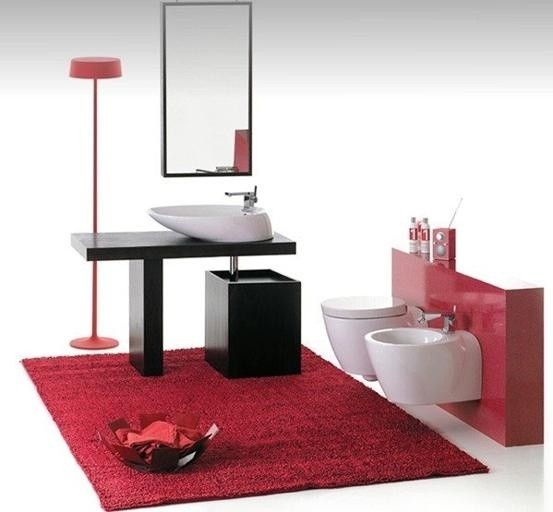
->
[146,204,274,242]
[364,325,483,406]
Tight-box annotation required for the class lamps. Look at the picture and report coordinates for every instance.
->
[69,55,123,351]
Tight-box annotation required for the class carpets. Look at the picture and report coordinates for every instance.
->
[22,343,491,510]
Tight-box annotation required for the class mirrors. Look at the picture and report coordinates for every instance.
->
[162,2,253,178]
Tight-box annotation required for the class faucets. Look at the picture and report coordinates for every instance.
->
[194,167,212,172]
[225,185,258,208]
[416,314,443,324]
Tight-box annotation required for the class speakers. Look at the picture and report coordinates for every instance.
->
[432,228,456,260]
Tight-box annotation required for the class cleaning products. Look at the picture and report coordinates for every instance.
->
[408,217,419,253]
[421,218,431,253]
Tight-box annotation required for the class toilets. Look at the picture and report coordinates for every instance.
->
[320,294,408,376]
